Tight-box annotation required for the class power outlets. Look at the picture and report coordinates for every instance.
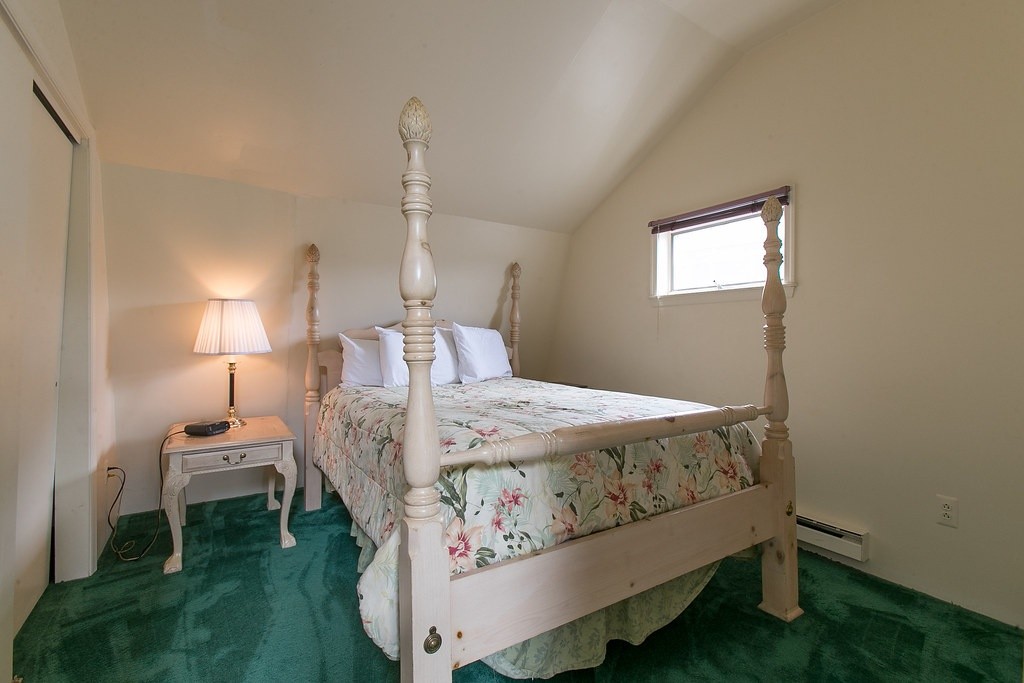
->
[935,494,960,529]
[104,459,109,485]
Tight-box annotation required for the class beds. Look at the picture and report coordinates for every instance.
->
[301,97,804,683]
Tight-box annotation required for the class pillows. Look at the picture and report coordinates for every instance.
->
[374,325,410,388]
[451,321,513,384]
[431,326,460,386]
[339,332,383,388]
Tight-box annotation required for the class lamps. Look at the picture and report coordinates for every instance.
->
[193,298,272,428]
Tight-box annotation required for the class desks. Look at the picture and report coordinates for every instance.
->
[161,415,297,575]
